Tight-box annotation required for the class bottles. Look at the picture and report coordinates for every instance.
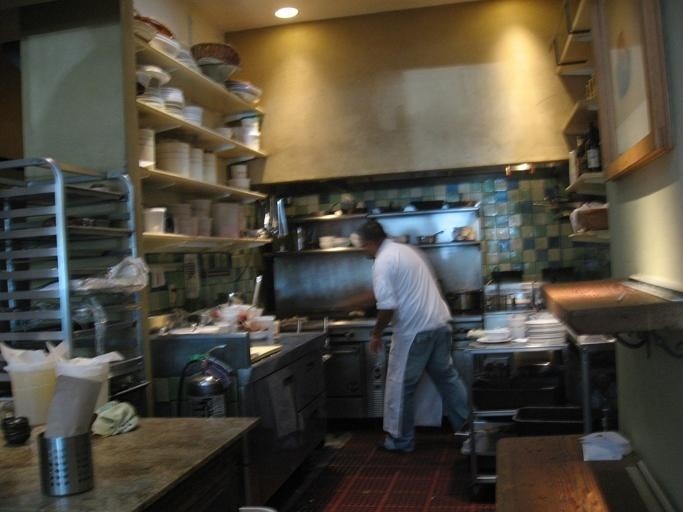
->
[584,120,601,171]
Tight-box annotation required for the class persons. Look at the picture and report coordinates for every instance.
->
[329,218,470,454]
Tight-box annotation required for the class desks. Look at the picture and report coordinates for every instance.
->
[1,414,261,511]
[493,434,675,512]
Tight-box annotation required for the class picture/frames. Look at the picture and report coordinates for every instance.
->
[591,0,671,182]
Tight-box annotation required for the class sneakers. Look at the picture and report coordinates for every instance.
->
[375,437,400,454]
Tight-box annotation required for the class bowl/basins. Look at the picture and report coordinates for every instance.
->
[513,407,597,435]
[319,233,360,247]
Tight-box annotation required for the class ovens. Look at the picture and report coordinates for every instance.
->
[328,341,366,396]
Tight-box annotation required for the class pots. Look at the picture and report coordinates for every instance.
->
[447,289,481,312]
[379,201,402,212]
[415,230,444,244]
[447,193,476,208]
[411,199,449,210]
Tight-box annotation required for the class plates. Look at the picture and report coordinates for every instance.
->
[477,314,566,347]
[134,16,261,237]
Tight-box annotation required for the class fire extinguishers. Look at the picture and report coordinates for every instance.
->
[176,344,234,417]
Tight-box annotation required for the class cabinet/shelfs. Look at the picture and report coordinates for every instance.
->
[464,330,571,499]
[0,156,157,420]
[534,1,609,240]
[271,200,482,258]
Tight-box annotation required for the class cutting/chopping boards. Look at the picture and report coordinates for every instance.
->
[250,346,281,361]
